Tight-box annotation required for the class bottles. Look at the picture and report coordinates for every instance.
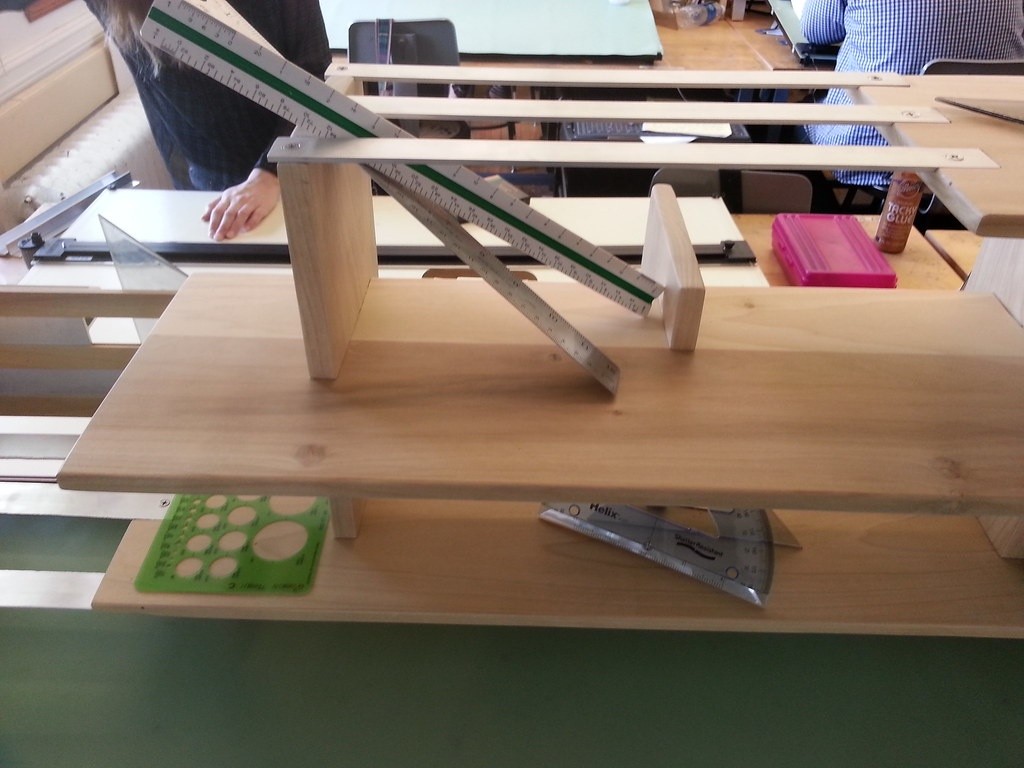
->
[676,2,726,30]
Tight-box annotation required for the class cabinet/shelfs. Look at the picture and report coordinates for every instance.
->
[57,49,1024,653]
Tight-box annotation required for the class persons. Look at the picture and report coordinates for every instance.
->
[86,0,333,242]
[804,0,1024,211]
